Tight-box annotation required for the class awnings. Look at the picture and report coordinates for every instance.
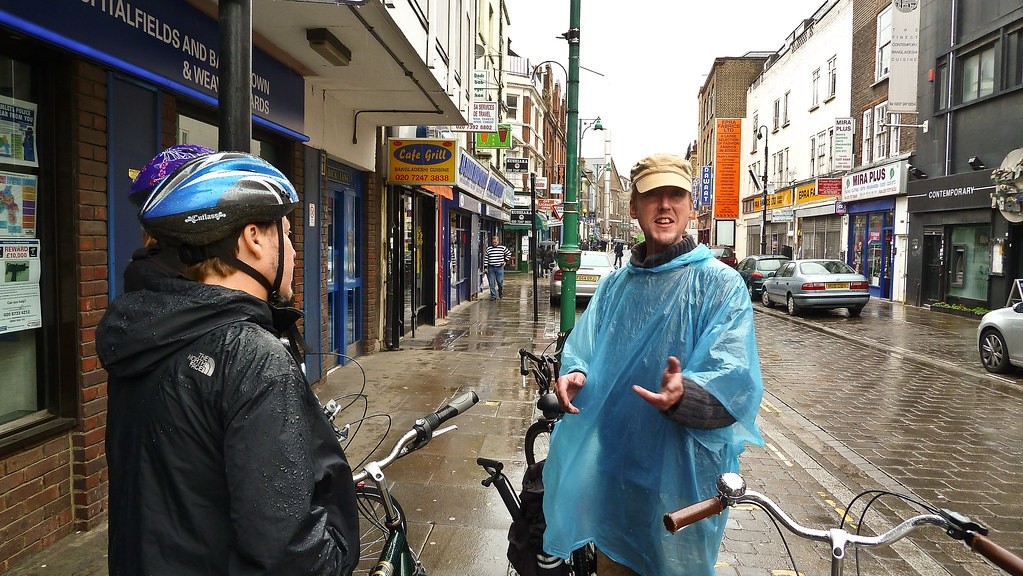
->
[536,213,547,231]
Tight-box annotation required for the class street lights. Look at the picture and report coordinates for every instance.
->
[757,124,769,256]
[577,116,604,248]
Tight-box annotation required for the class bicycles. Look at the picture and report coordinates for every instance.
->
[663,472,1023,576]
[477,328,599,576]
[298,351,478,576]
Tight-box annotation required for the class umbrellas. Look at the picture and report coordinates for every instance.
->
[539,241,555,245]
[613,239,626,243]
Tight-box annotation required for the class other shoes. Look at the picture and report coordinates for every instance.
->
[499,294,503,298]
[491,297,496,301]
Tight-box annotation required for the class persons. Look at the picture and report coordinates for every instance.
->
[537,242,559,279]
[580,238,608,253]
[485,236,512,300]
[544,156,763,576]
[95,144,361,576]
[628,240,639,250]
[614,242,622,268]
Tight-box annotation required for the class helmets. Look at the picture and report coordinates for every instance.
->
[125,145,219,200]
[137,150,301,246]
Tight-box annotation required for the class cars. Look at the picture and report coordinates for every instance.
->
[761,259,870,316]
[735,254,790,301]
[549,250,616,306]
[977,301,1023,376]
[708,246,739,268]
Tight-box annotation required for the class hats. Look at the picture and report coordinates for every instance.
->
[629,153,694,195]
